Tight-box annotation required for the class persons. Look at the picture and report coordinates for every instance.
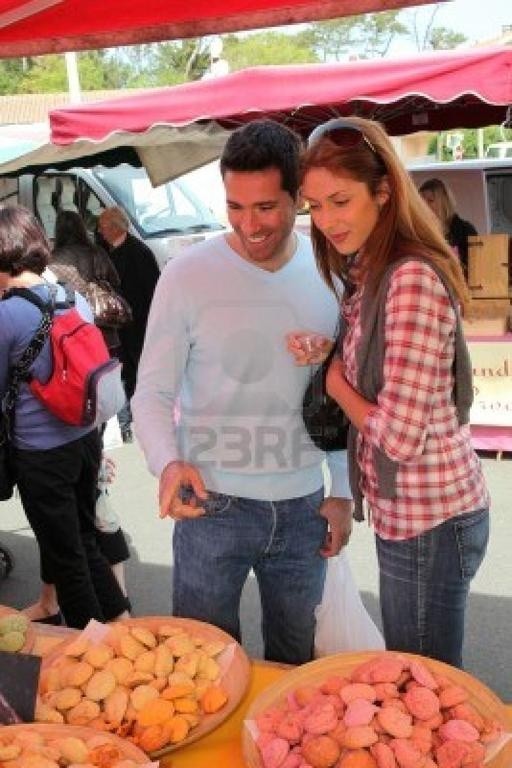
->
[283,112,491,673]
[416,177,480,280]
[1,199,164,634]
[129,116,358,666]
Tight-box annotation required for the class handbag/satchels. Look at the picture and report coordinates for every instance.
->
[298,305,353,452]
[83,273,136,331]
[1,386,19,502]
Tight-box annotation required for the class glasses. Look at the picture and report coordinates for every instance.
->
[305,120,386,166]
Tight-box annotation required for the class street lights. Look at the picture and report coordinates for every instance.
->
[210,36,224,63]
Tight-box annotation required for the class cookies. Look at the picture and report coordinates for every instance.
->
[1,612,503,768]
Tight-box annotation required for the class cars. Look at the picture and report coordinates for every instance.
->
[18,163,235,273]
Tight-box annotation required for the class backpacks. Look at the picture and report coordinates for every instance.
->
[0,279,128,430]
